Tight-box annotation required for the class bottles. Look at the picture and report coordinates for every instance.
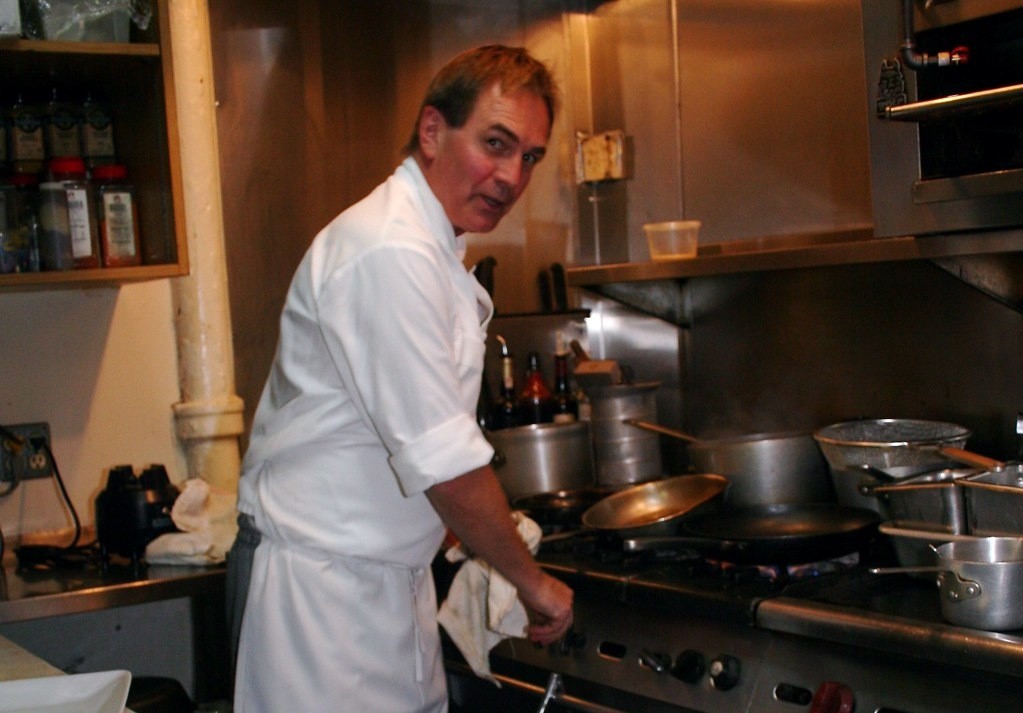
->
[495,353,519,429]
[0,72,141,273]
[550,352,576,424]
[521,356,551,425]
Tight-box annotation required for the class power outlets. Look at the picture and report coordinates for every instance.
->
[0,423,52,481]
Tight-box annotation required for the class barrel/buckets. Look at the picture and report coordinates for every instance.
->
[576,381,663,486]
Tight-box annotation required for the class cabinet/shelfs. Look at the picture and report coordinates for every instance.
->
[0,0,190,286]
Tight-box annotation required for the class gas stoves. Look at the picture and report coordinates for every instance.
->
[505,537,867,689]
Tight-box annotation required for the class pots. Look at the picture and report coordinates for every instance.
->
[580,473,731,534]
[869,536,1023,631]
[624,500,881,566]
[485,421,587,499]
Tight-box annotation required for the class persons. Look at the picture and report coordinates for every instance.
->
[232,42,573,713]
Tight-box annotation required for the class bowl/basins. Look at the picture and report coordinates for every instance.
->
[685,430,817,502]
[811,416,972,513]
[643,221,701,260]
[873,464,1023,575]
